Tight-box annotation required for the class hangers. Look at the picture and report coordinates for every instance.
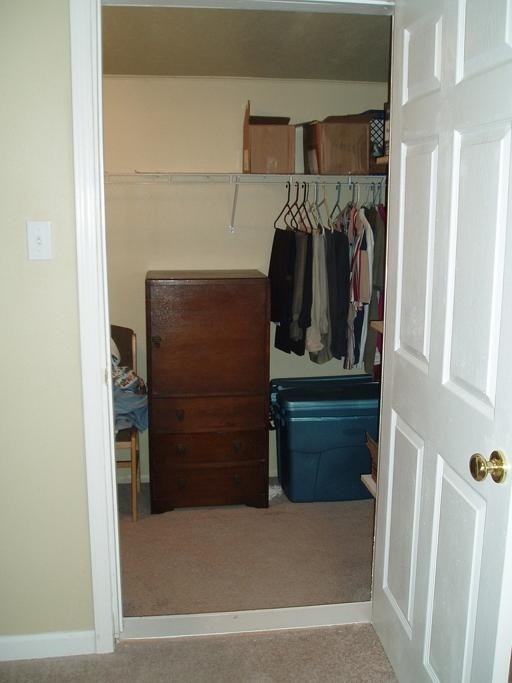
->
[273,181,386,238]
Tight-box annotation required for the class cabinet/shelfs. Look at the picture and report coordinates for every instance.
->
[145,269,271,516]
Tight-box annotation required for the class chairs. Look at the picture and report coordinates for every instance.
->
[110,326,141,522]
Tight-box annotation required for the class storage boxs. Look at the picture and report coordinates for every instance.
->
[268,373,379,503]
[242,100,370,176]
